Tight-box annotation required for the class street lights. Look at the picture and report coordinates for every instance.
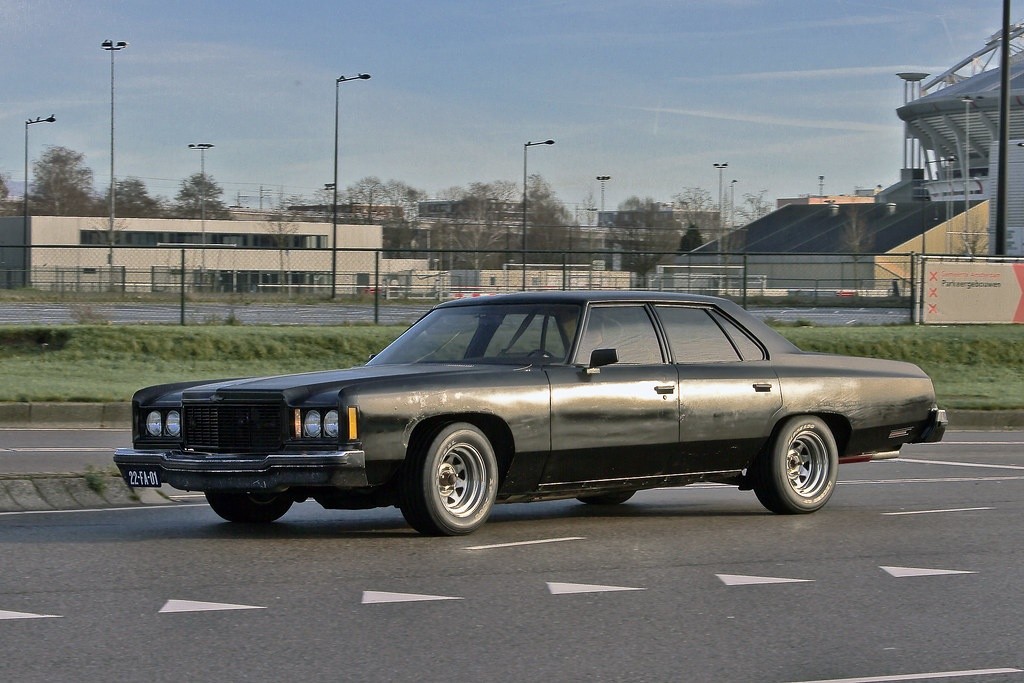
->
[596,175,611,249]
[714,163,728,217]
[98,38,128,293]
[959,95,982,252]
[331,73,371,300]
[520,140,555,292]
[187,142,214,270]
[23,112,57,288]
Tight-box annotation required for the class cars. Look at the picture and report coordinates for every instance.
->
[112,288,948,538]
